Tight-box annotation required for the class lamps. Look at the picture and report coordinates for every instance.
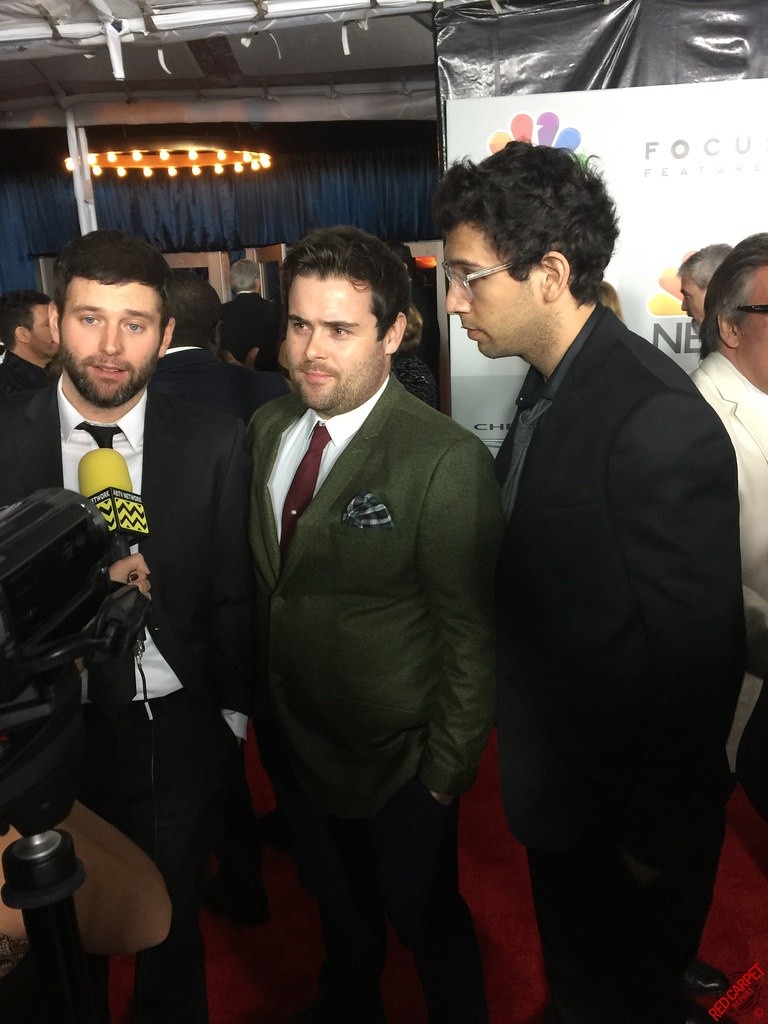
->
[62,123,273,176]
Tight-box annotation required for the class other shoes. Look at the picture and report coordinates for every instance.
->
[201,875,273,925]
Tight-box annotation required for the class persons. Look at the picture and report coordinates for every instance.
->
[0,146,767,1022]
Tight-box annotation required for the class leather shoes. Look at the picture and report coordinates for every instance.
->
[701,966,730,997]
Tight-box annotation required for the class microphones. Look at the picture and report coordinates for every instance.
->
[79,447,150,566]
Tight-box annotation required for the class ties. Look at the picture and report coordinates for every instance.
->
[498,398,551,524]
[75,422,123,449]
[279,421,331,564]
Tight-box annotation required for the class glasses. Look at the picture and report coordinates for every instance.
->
[441,243,561,300]
